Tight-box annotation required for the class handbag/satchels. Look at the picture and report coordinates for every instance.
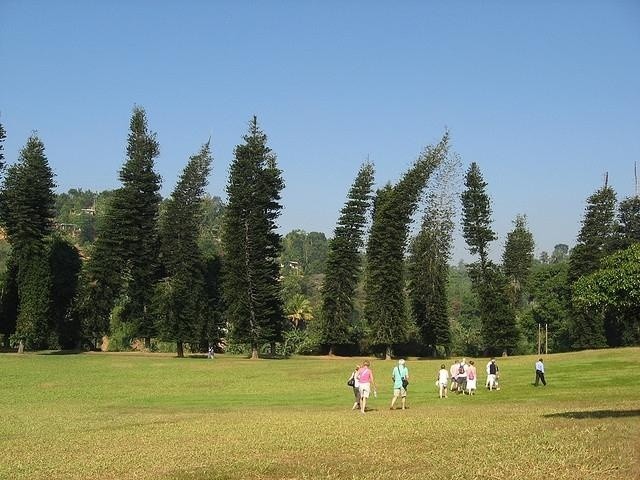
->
[348,379,354,386]
[490,365,498,374]
[402,377,408,388]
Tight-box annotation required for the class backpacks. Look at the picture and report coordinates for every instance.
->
[459,363,465,374]
[469,366,474,380]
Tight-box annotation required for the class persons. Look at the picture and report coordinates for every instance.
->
[535,358,546,387]
[390,359,410,410]
[437,365,449,398]
[451,358,476,396]
[348,360,376,413]
[485,357,500,391]
